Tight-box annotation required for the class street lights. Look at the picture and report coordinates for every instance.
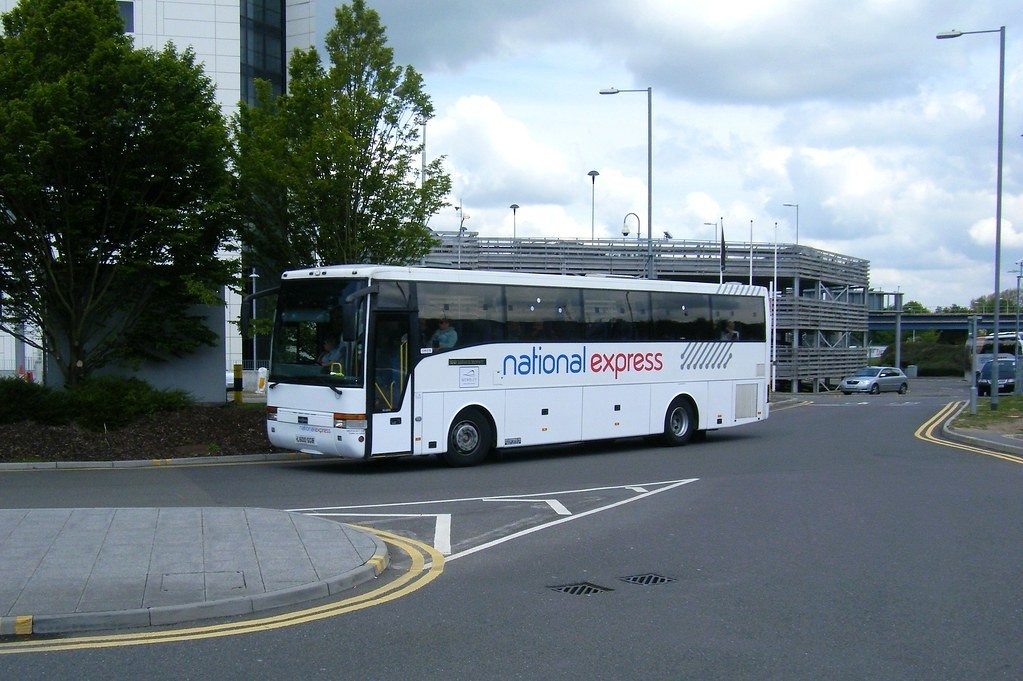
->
[935,26,1006,411]
[704,223,718,258]
[599,86,653,260]
[510,204,520,243]
[908,308,916,344]
[975,301,986,315]
[249,268,259,391]
[1000,298,1014,312]
[588,171,600,246]
[783,203,799,245]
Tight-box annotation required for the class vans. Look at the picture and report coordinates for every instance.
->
[976,358,1017,397]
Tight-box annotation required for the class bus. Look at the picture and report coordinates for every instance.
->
[241,263,772,468]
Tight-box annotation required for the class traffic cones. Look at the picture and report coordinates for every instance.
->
[18,365,25,379]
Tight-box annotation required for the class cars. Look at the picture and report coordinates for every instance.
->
[841,367,908,395]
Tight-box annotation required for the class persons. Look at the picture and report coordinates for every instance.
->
[428,316,458,351]
[720,320,739,340]
[320,337,346,373]
